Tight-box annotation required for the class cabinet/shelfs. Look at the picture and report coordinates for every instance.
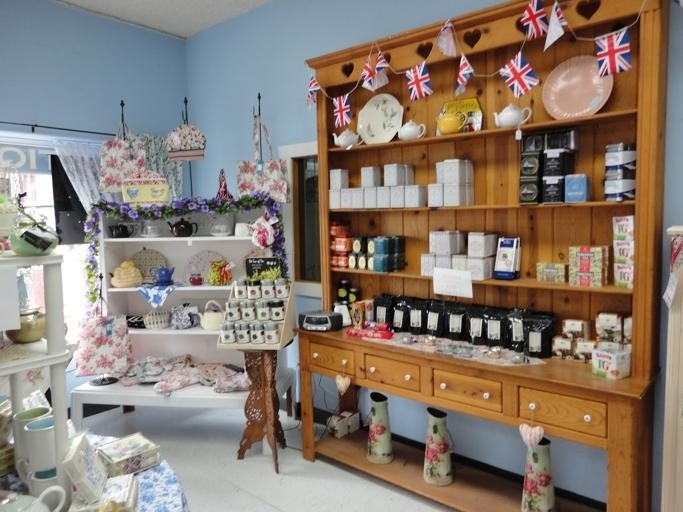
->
[293,0,670,512]
[70,196,294,476]
[0,249,73,511]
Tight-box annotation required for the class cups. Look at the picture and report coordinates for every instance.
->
[13,407,71,512]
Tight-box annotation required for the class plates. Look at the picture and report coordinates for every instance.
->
[357,93,403,144]
[541,55,613,120]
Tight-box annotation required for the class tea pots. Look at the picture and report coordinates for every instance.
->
[166,217,198,237]
[108,222,135,237]
[332,129,365,147]
[493,103,532,126]
[197,300,227,331]
[150,266,175,287]
[434,110,468,134]
[392,119,427,140]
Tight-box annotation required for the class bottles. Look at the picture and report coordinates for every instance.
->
[221,278,289,344]
[365,391,393,464]
[520,438,556,511]
[189,273,203,285]
[422,406,451,486]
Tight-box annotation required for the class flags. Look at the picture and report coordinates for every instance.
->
[404,25,474,102]
[594,28,633,78]
[307,51,389,128]
[499,0,568,99]
